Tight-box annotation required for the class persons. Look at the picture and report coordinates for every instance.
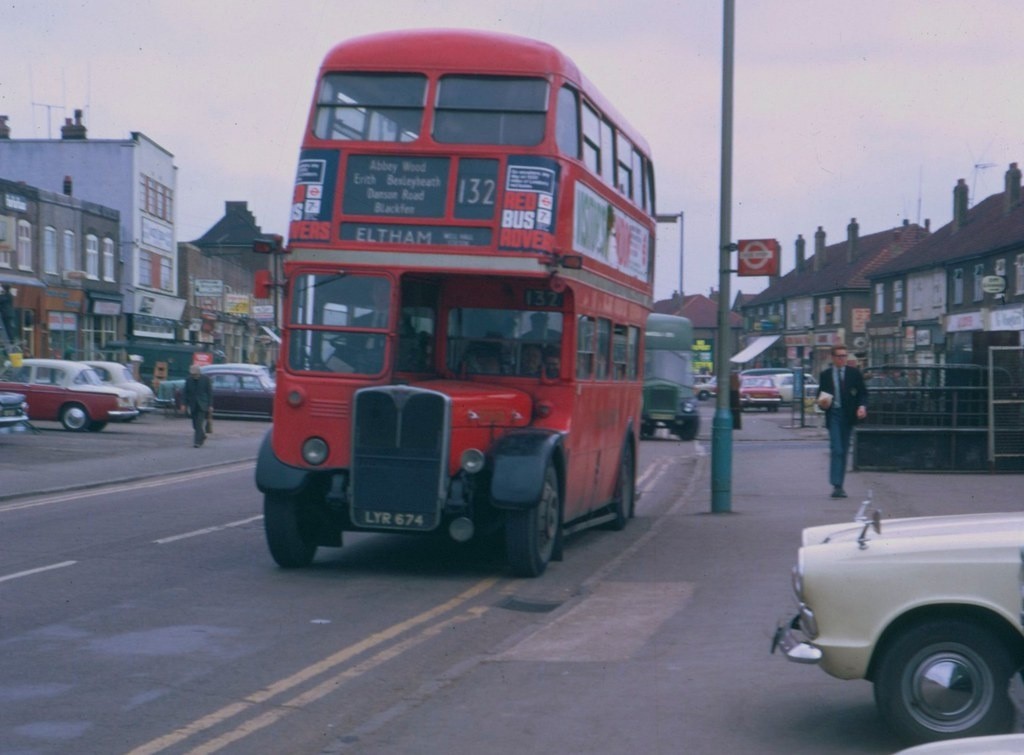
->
[180,365,213,448]
[817,346,866,498]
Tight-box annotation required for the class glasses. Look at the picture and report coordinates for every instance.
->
[545,362,559,366]
[833,354,848,358]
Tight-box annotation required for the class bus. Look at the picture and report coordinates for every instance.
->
[640,310,711,441]
[248,24,661,580]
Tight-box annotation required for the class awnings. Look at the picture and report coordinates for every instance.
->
[730,336,782,363]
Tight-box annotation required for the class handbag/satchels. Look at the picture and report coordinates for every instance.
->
[843,396,865,424]
[205,412,214,433]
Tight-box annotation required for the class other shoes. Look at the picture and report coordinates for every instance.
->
[200,435,207,445]
[193,443,201,448]
[830,487,849,499]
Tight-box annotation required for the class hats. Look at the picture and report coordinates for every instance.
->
[530,312,550,321]
[189,365,201,375]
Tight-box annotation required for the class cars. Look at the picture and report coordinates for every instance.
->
[766,485,1024,742]
[693,367,820,414]
[0,355,275,435]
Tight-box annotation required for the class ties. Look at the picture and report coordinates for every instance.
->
[374,313,384,340]
[837,369,845,408]
